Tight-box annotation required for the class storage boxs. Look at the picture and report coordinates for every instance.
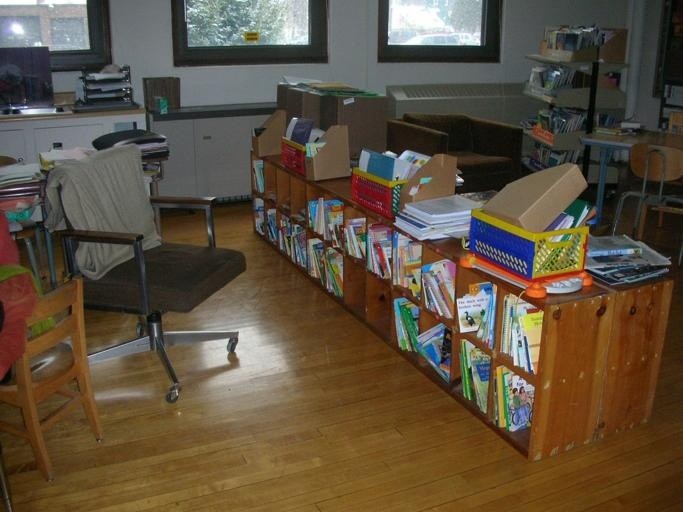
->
[251,79,390,184]
[477,164,587,230]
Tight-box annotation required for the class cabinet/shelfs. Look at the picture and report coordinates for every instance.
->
[523,26,632,190]
[248,149,678,465]
[146,101,281,219]
[0,104,145,167]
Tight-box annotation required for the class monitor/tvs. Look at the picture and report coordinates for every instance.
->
[0,45,56,116]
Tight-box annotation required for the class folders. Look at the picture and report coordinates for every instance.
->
[533,198,597,273]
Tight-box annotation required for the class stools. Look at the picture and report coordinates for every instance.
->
[12,224,43,276]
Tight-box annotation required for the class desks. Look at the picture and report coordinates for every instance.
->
[583,125,682,231]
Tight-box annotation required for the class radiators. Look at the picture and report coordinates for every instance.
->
[385,83,539,132]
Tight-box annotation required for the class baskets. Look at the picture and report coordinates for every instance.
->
[279,135,306,177]
[469,208,590,288]
[351,167,432,219]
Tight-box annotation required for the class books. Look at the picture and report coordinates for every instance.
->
[391,194,483,242]
[391,227,424,301]
[353,146,467,189]
[343,209,367,261]
[456,339,492,418]
[0,148,94,231]
[412,320,453,385]
[305,196,325,235]
[252,198,266,237]
[540,21,614,51]
[583,240,672,288]
[518,104,640,141]
[251,156,266,194]
[586,233,643,258]
[491,364,536,434]
[91,132,171,180]
[365,220,392,285]
[72,70,129,104]
[305,235,324,283]
[420,258,458,323]
[278,213,291,257]
[393,296,422,356]
[279,75,384,98]
[324,246,343,300]
[266,208,278,246]
[500,292,546,376]
[524,64,620,91]
[455,278,498,351]
[323,198,343,247]
[281,117,326,174]
[528,142,582,172]
[290,222,306,270]
[532,198,598,276]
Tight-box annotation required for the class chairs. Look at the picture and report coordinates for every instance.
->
[667,111,682,132]
[611,139,682,250]
[1,274,105,487]
[43,140,245,410]
[388,110,526,192]
